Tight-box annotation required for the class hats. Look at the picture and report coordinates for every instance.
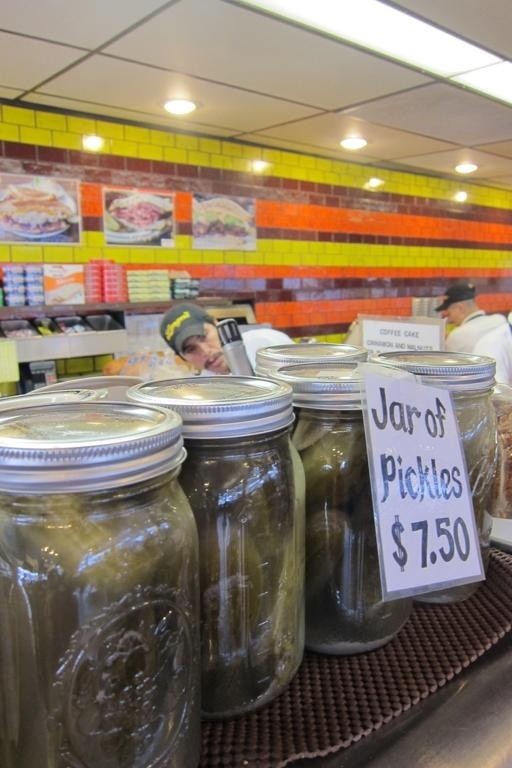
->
[435,284,475,311]
[159,302,210,354]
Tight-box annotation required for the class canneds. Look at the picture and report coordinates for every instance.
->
[0,399,202,768]
[126,374,307,721]
[371,349,497,605]
[268,360,413,655]
[254,343,369,378]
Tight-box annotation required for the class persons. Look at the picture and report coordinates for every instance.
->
[161,302,299,380]
[433,279,504,355]
[472,308,512,388]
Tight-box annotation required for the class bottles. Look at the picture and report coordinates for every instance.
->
[250,343,496,658]
[1,343,309,763]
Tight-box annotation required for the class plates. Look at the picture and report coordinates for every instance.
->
[2,183,172,245]
